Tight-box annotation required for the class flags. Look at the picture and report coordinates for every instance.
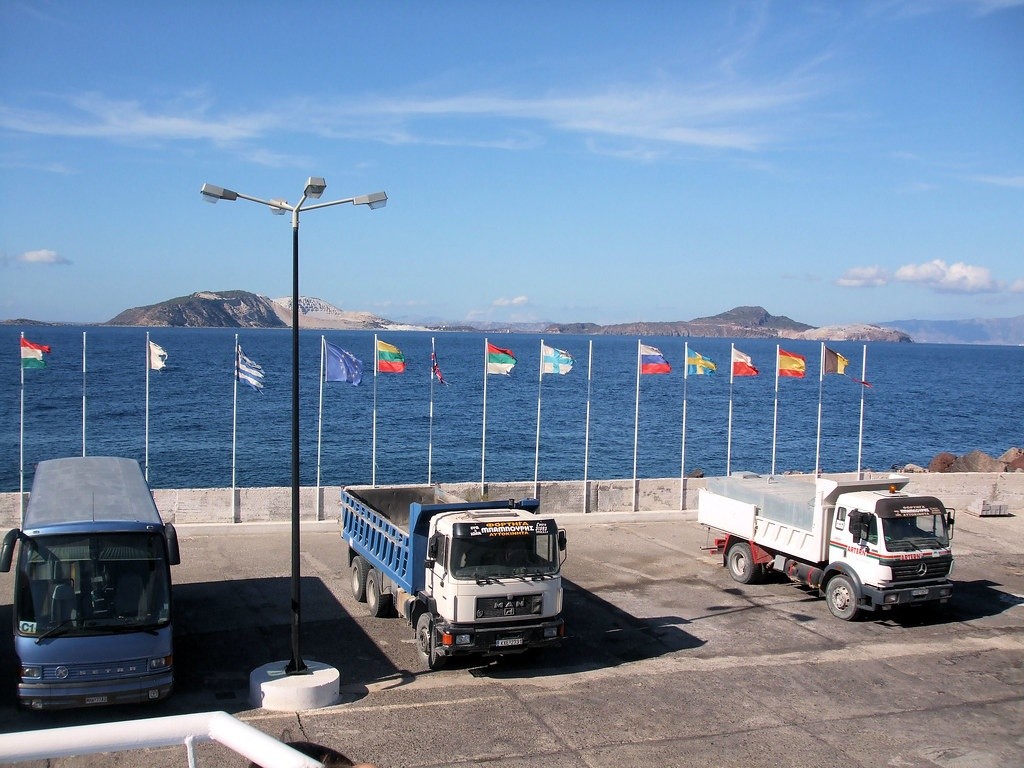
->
[683,347,717,377]
[232,344,265,396]
[637,345,671,374]
[484,343,518,374]
[374,339,406,373]
[731,348,760,377]
[538,344,573,374]
[146,340,168,372]
[19,337,52,370]
[823,346,872,388]
[324,340,363,386]
[430,346,453,386]
[776,345,807,379]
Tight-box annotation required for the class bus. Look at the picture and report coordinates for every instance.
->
[1,456,181,720]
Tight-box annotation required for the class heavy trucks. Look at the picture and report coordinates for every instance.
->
[335,484,570,676]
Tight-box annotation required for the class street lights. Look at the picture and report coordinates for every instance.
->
[198,173,388,724]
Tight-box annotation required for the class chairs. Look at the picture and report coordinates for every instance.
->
[508,548,532,566]
[50,585,74,622]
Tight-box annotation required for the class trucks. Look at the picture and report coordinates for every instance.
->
[695,473,956,621]
